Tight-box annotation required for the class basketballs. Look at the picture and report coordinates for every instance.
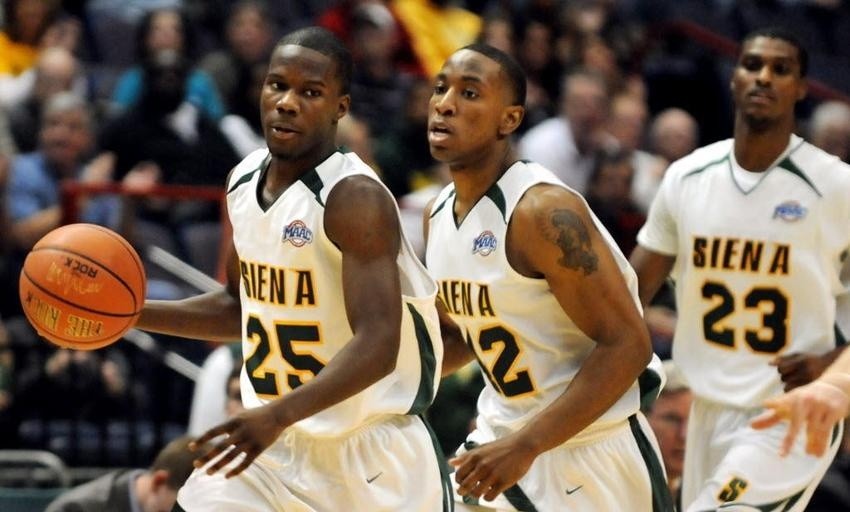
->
[19,223,148,351]
